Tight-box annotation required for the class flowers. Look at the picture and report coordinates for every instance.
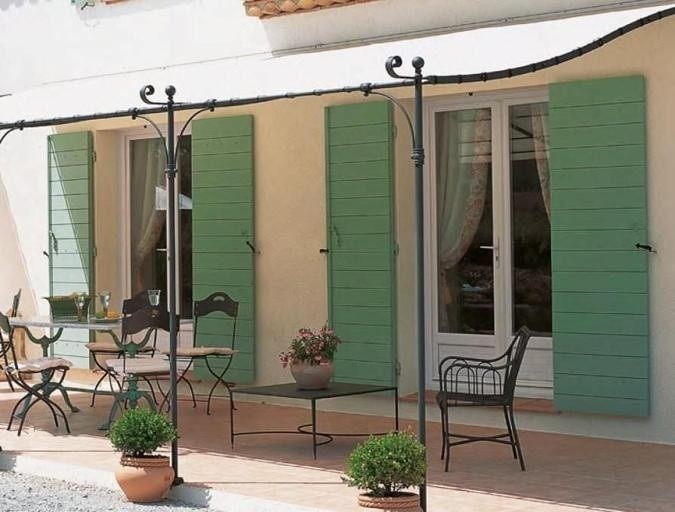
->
[279,327,345,368]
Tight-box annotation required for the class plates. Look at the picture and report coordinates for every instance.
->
[89,311,124,323]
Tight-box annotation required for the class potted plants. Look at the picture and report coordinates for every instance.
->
[345,426,427,511]
[105,407,180,503]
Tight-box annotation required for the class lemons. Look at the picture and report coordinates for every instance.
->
[96,311,105,319]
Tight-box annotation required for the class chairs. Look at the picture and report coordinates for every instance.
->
[437,324,533,473]
[0,288,241,435]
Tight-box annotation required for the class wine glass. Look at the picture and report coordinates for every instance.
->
[97,288,112,311]
[147,289,162,315]
[73,291,87,323]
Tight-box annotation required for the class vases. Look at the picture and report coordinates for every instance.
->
[291,361,332,390]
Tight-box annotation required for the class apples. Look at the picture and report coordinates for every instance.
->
[107,312,119,318]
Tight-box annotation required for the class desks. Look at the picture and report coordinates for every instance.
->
[225,382,400,459]
